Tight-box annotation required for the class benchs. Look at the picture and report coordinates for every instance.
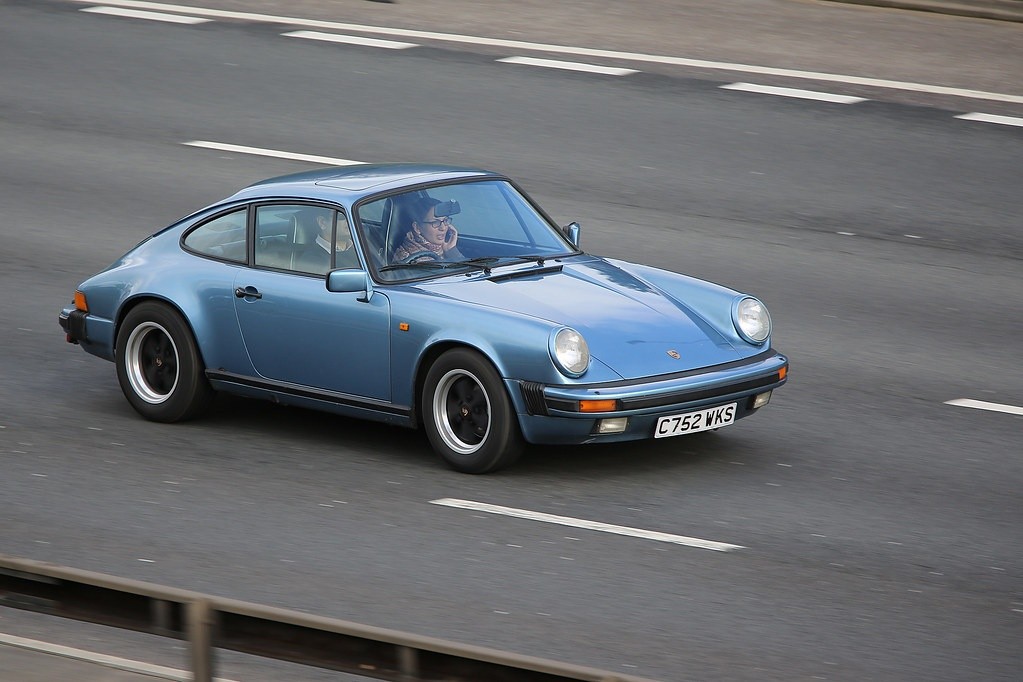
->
[208,232,289,269]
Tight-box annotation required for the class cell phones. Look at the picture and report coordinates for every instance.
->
[444,228,452,243]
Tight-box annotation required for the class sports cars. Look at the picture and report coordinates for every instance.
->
[60,165,787,474]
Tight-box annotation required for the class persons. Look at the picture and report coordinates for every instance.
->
[391,198,464,264]
[296,208,360,274]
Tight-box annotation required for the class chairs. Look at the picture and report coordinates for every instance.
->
[283,210,322,270]
[379,191,418,265]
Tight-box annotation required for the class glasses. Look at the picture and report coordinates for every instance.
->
[422,217,452,228]
[328,214,346,220]
